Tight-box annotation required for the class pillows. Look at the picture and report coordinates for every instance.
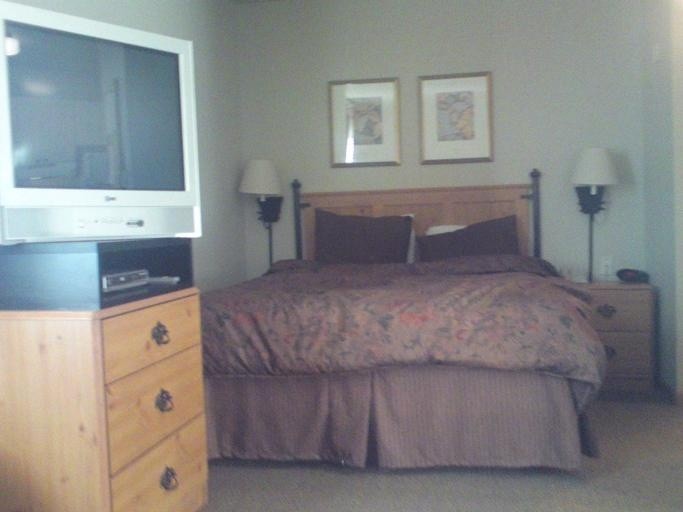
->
[315,205,519,266]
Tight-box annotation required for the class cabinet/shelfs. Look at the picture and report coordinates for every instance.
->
[0,288,209,512]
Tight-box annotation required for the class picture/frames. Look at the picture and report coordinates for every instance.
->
[327,77,402,167]
[416,72,496,164]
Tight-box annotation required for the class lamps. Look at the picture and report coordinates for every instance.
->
[237,157,280,266]
[570,146,619,282]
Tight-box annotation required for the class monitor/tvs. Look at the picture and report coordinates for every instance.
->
[0,1,203,246]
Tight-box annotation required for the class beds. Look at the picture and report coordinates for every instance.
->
[197,167,606,478]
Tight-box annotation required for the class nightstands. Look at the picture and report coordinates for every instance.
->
[565,275,657,403]
